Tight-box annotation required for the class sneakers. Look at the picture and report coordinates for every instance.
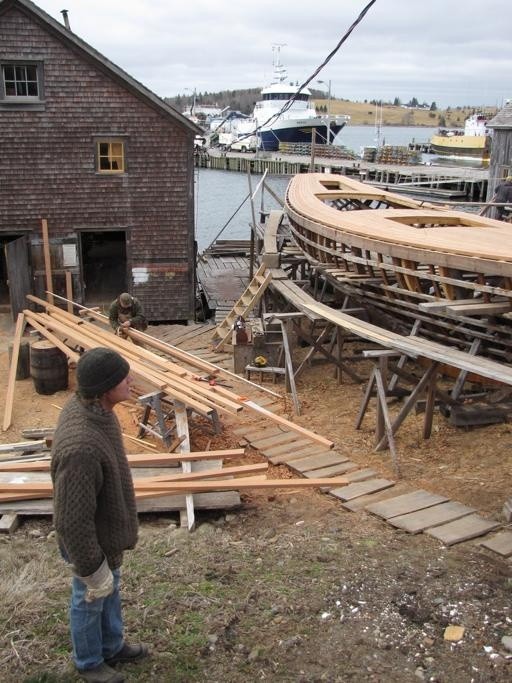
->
[106,642,147,664]
[79,664,126,683]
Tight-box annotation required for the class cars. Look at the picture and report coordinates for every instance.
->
[228,135,261,152]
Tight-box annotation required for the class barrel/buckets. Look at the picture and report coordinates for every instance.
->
[31,339,69,396]
[8,341,30,380]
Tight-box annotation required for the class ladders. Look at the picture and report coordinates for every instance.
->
[211,263,272,352]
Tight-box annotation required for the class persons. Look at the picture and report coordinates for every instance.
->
[50,347,155,683]
[494,175,512,220]
[108,292,147,348]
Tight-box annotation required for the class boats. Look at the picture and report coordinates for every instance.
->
[183,103,231,125]
[430,112,492,157]
[209,42,350,151]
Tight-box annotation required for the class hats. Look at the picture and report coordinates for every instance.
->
[77,348,129,398]
[120,293,132,308]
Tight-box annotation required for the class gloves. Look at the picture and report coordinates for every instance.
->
[75,552,113,603]
